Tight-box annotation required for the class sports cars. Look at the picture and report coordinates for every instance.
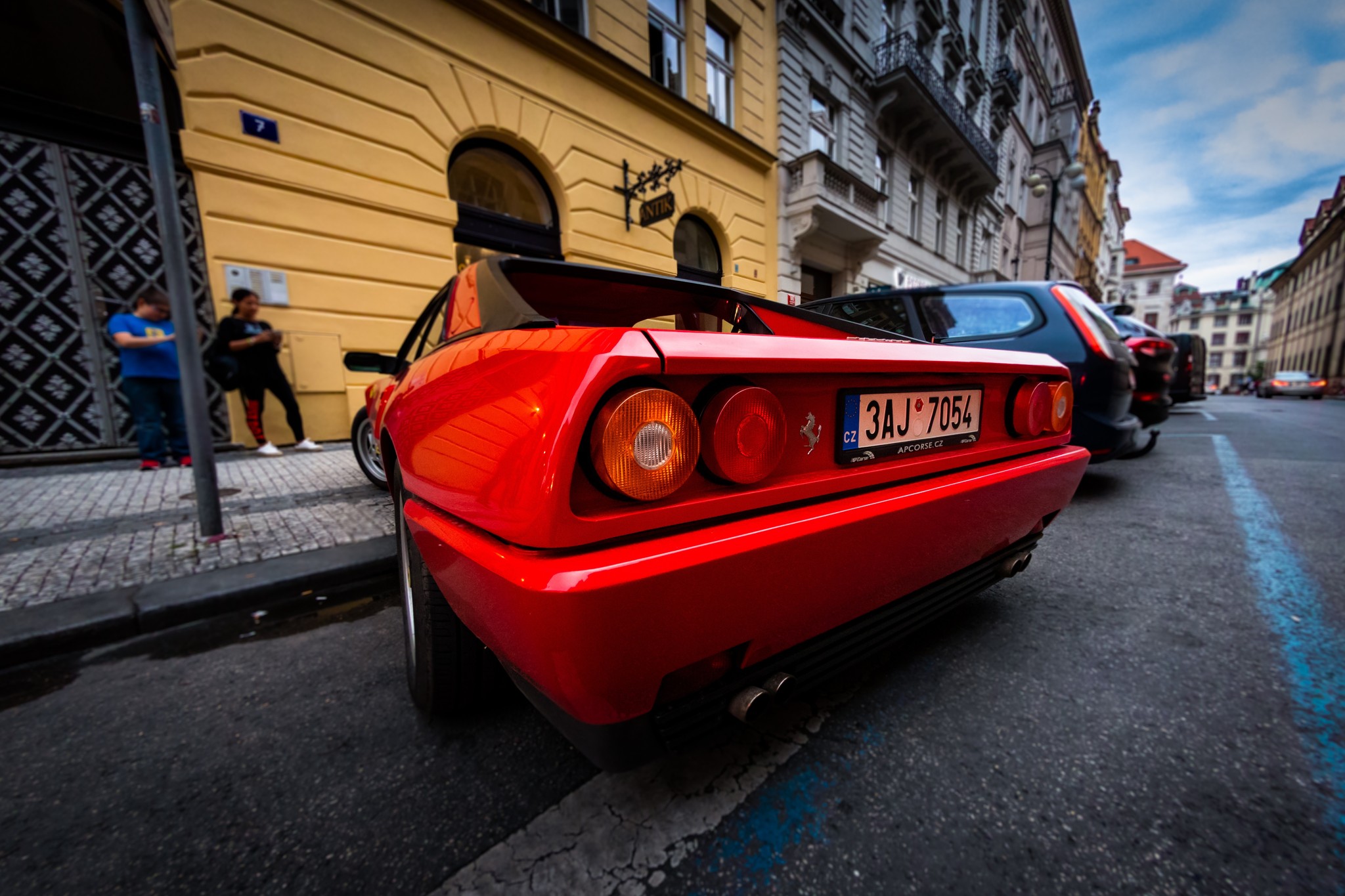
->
[341,255,1095,731]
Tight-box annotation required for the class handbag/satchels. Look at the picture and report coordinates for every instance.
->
[201,336,238,392]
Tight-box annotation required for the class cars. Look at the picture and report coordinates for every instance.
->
[1256,369,1325,399]
[1204,380,1218,393]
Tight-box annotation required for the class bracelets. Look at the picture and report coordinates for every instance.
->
[247,337,253,346]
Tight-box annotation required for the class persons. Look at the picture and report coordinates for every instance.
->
[108,287,192,471]
[217,287,323,455]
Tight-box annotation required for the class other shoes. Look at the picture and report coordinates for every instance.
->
[294,437,324,452]
[138,460,162,471]
[179,456,193,468]
[256,440,284,457]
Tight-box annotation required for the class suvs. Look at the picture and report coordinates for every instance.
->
[1098,297,1180,431]
[1164,331,1212,404]
[790,278,1147,464]
